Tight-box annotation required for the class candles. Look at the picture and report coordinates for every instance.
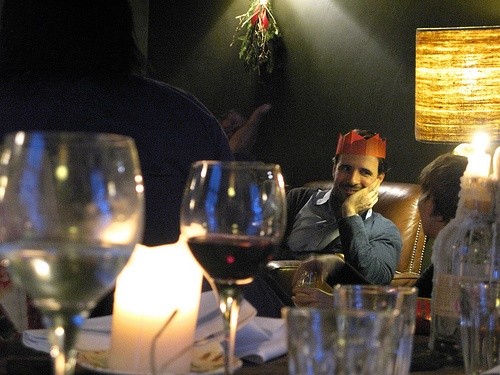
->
[465,155,492,176]
[109,243,203,374]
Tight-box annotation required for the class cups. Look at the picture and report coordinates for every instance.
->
[286,310,378,375]
[458,279,500,375]
[334,286,418,375]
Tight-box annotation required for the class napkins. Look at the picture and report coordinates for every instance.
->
[24,289,290,364]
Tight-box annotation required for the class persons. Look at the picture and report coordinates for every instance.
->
[267,129,403,287]
[288,152,467,310]
[0,0,296,318]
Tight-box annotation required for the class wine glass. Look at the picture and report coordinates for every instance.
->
[181,161,288,375]
[0,130,145,375]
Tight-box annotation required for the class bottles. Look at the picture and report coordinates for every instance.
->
[430,178,491,350]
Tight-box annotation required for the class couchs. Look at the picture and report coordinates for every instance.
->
[263,182,426,298]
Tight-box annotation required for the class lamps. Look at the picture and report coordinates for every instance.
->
[415,25,500,146]
